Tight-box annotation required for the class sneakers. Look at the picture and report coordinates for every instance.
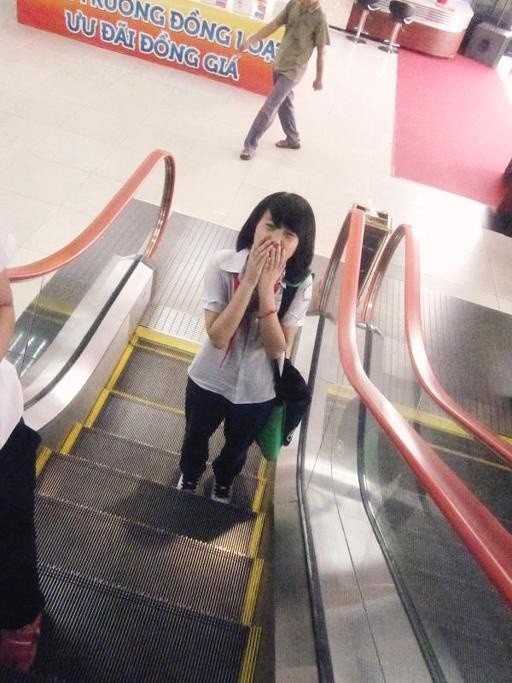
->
[211,470,234,503]
[241,146,257,160]
[176,472,203,495]
[1,613,42,677]
[275,139,299,147]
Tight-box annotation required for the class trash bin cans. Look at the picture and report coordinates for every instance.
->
[351,204,392,301]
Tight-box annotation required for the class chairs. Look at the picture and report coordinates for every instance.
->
[346,0,416,56]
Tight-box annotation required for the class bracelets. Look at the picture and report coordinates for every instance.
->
[258,306,277,320]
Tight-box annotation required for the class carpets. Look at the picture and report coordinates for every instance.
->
[393,42,510,208]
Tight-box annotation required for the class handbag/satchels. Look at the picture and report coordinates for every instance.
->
[256,405,284,465]
[276,359,312,446]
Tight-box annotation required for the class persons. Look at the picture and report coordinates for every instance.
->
[0,252,43,677]
[176,192,316,506]
[236,0,332,160]
